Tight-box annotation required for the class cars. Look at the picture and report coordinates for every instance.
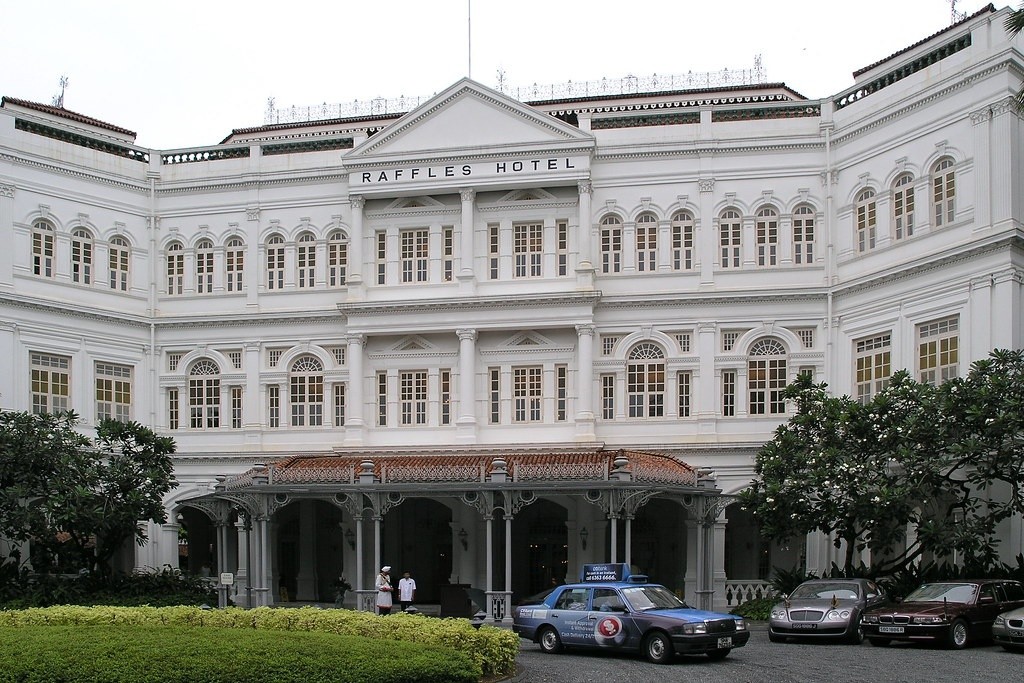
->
[861,579,1024,648]
[991,608,1024,649]
[768,578,886,644]
[517,560,751,665]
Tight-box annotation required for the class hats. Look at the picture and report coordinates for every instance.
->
[381,566,391,572]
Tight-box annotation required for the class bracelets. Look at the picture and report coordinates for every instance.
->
[399,595,400,596]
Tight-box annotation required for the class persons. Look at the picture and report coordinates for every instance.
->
[397,571,416,612]
[599,593,622,612]
[376,566,394,615]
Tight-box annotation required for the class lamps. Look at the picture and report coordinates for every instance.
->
[580,527,588,550]
[201,603,211,610]
[458,528,468,551]
[405,605,418,614]
[314,604,322,609]
[471,618,483,630]
[345,528,355,551]
[473,610,488,621]
